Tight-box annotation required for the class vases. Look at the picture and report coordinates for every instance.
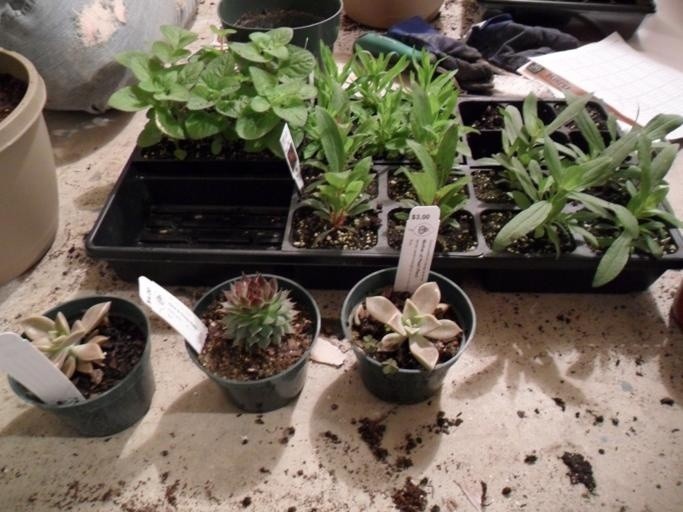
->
[216,0,347,78]
[0,43,61,286]
[183,274,321,414]
[342,0,445,32]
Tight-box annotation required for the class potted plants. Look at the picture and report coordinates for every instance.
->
[6,294,157,437]
[340,266,477,406]
